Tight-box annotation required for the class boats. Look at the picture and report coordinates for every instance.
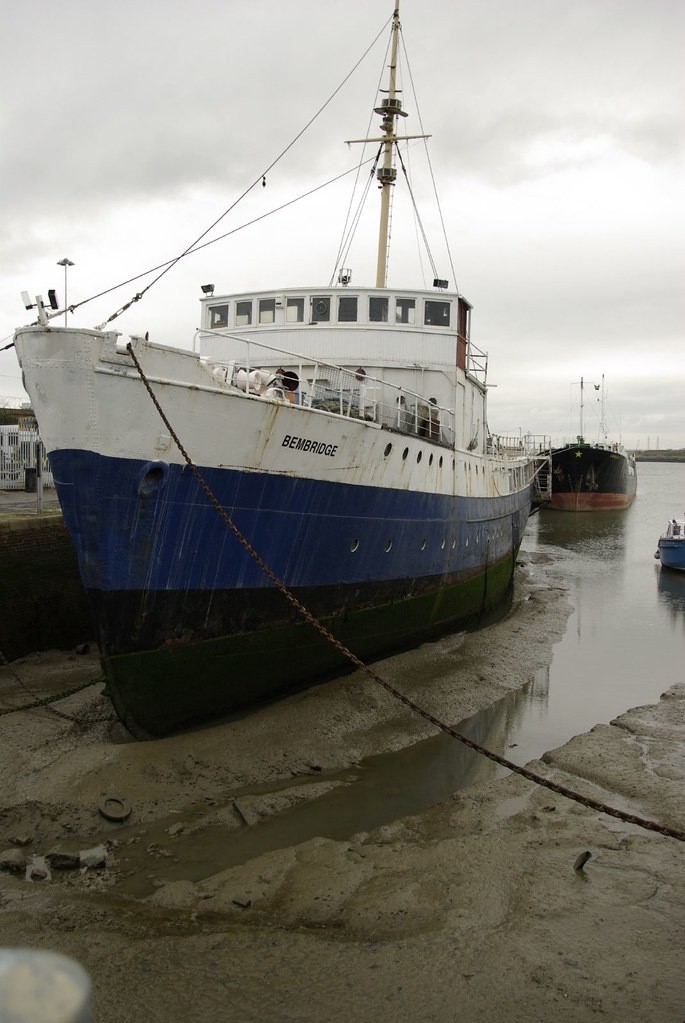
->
[17,0,540,740]
[655,518,685,572]
[534,374,638,511]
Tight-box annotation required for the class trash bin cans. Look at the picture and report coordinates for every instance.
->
[25,467,37,493]
[285,391,307,406]
